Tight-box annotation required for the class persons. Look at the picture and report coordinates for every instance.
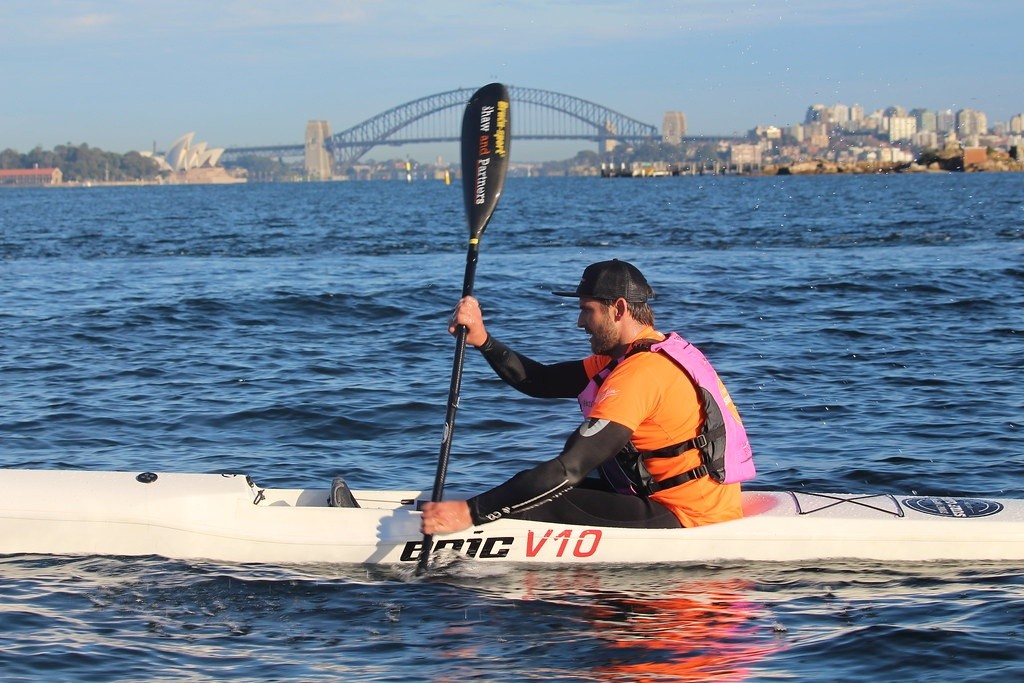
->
[330,258,744,535]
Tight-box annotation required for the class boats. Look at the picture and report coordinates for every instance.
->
[0,465,1024,576]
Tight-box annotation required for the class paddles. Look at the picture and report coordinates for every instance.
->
[413,82,513,577]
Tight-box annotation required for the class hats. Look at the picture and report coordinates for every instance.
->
[552,258,648,303]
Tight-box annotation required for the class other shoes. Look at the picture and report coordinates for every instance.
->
[329,476,360,508]
